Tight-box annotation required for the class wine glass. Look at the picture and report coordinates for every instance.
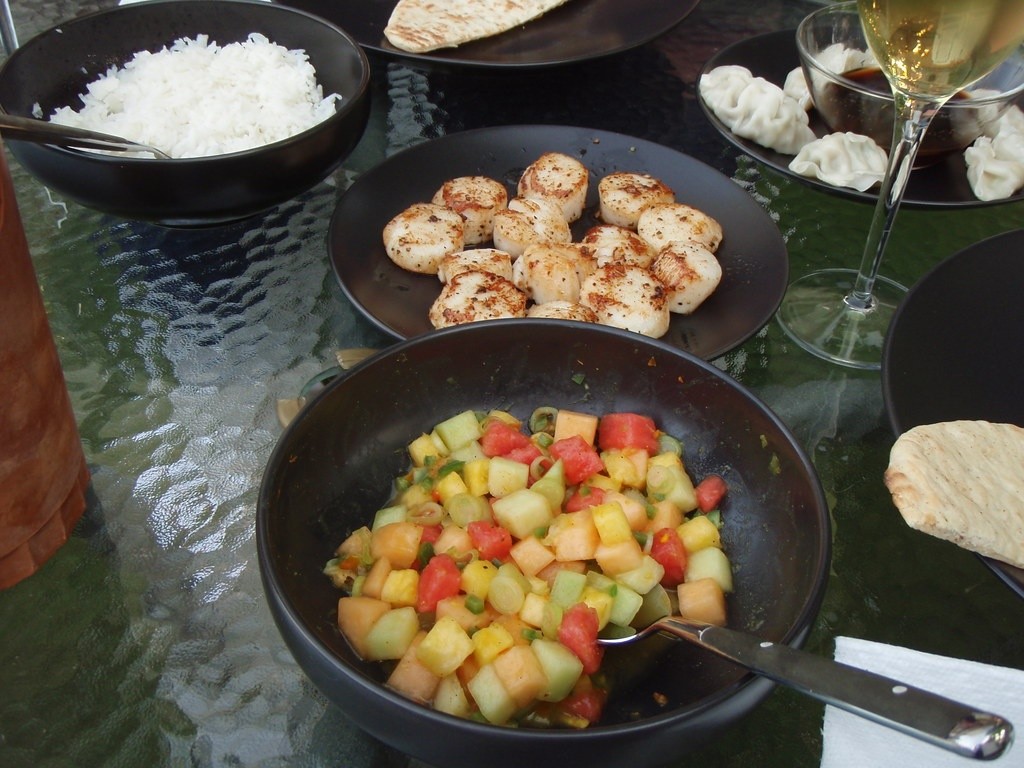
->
[772,0,1024,370]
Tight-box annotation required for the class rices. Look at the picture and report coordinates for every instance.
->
[31,32,344,158]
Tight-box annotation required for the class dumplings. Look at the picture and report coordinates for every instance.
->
[699,42,1024,201]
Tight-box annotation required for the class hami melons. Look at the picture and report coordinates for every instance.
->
[332,408,727,705]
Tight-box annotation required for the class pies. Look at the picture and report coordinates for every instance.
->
[382,0,569,53]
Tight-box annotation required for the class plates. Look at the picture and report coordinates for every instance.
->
[880,230,1024,601]
[326,125,790,362]
[272,0,700,72]
[697,28,1024,210]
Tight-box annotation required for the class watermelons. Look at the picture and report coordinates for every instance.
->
[415,413,726,722]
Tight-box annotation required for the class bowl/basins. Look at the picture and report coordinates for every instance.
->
[255,308,833,768]
[796,0,1024,172]
[0,0,373,232]
